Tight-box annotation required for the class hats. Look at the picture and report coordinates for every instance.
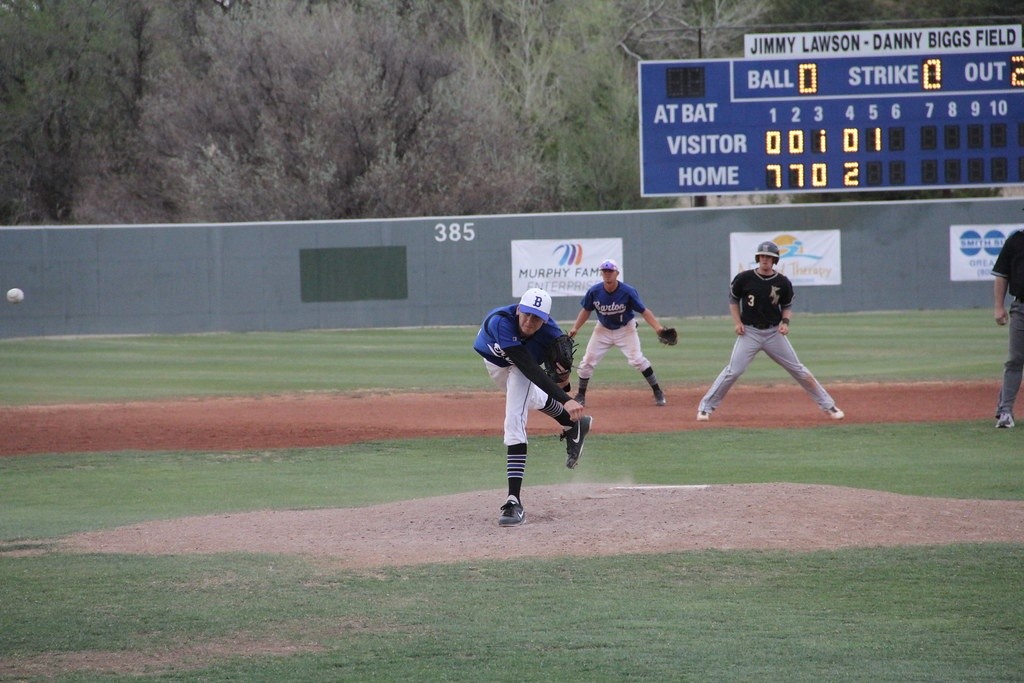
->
[519,288,552,321]
[598,259,618,272]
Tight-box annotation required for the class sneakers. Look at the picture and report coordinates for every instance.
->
[995,413,1015,428]
[696,411,709,421]
[826,406,844,420]
[560,416,592,469]
[498,500,526,527]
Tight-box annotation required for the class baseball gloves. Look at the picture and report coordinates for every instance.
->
[659,328,678,346]
[545,335,575,383]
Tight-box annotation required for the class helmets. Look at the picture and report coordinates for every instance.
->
[755,241,779,265]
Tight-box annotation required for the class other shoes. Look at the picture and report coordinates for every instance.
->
[573,397,584,407]
[653,389,665,406]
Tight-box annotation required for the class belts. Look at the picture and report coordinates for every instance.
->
[1014,296,1024,303]
[744,323,777,329]
[603,323,627,330]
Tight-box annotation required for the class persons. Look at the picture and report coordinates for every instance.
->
[991,207,1024,428]
[696,241,844,421]
[473,288,592,527]
[568,259,677,409]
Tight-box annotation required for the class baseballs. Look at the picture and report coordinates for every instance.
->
[7,288,23,303]
